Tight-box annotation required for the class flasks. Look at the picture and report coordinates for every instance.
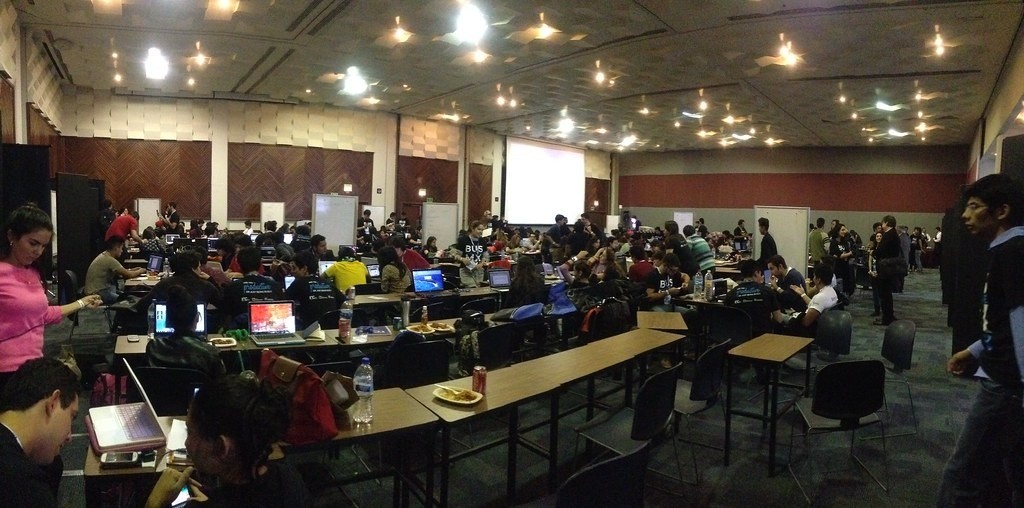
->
[400,297,410,329]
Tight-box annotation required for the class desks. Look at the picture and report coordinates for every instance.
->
[81,247,814,508]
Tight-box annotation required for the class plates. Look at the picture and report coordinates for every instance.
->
[206,337,237,347]
[406,324,436,334]
[427,322,451,332]
[433,386,484,404]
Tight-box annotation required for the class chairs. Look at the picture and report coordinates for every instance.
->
[59,271,915,508]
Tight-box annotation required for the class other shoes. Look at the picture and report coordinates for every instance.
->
[870,311,880,316]
[687,350,702,360]
[874,319,889,326]
[661,357,672,369]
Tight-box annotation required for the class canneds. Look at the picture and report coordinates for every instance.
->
[392,317,402,330]
[737,255,741,263]
[339,319,350,337]
[348,287,355,299]
[472,366,487,395]
[704,286,713,301]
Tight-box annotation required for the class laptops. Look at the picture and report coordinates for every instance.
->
[367,264,381,281]
[88,358,168,450]
[339,245,357,257]
[206,238,219,252]
[166,234,180,244]
[488,269,511,292]
[556,266,566,280]
[284,275,296,291]
[250,234,258,243]
[318,261,337,281]
[764,270,772,283]
[284,233,293,245]
[140,255,162,276]
[411,269,461,299]
[248,300,306,347]
[154,301,208,343]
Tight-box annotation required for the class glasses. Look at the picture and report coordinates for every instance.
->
[965,204,989,211]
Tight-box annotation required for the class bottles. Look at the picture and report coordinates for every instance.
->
[147,298,157,341]
[693,270,703,302]
[421,305,428,326]
[338,298,352,343]
[705,270,714,302]
[353,356,374,424]
[663,294,671,305]
[712,246,715,258]
[164,258,170,279]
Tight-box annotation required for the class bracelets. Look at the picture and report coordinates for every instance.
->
[77,299,85,311]
[666,290,669,295]
[571,256,578,262]
[776,287,781,291]
[801,293,806,297]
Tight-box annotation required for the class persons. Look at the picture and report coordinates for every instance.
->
[85,236,147,333]
[0,357,79,508]
[146,287,226,377]
[723,219,841,384]
[445,211,748,368]
[940,185,987,377]
[936,174,1024,508]
[145,376,314,508]
[0,202,102,388]
[102,200,438,334]
[809,215,942,325]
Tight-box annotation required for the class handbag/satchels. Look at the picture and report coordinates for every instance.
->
[259,349,336,447]
[90,373,128,404]
[601,297,630,336]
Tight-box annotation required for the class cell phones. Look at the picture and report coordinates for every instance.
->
[100,451,137,465]
[127,335,139,342]
[169,482,192,508]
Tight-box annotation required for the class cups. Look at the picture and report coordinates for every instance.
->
[348,287,355,300]
